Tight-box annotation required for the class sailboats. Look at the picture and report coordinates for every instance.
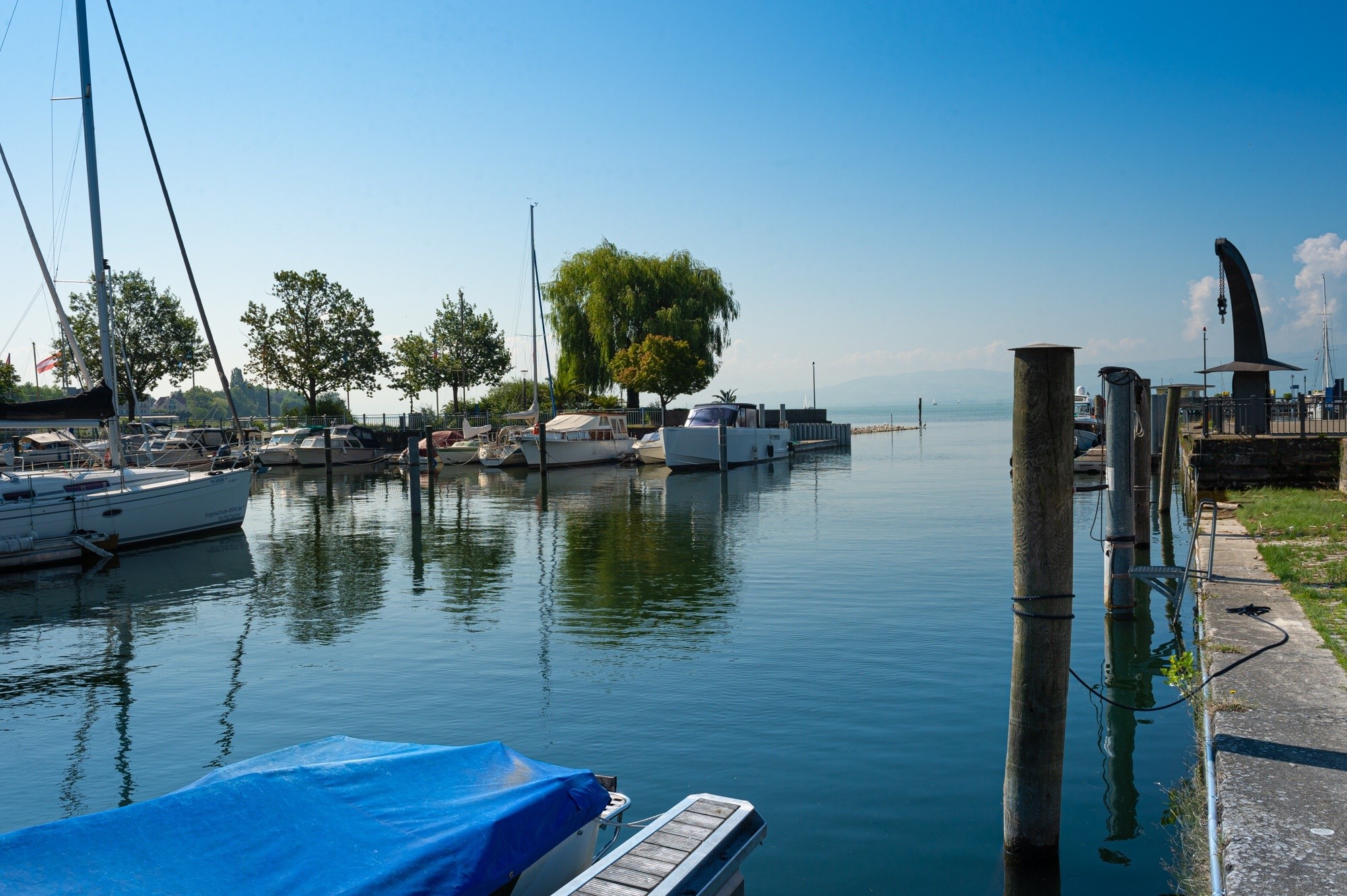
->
[505,197,641,469]
[0,0,253,565]
[932,398,938,405]
[1308,274,1342,420]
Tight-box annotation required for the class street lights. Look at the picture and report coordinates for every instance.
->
[520,371,528,410]
[1290,374,1294,401]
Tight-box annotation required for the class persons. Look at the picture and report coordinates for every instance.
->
[59,462,89,473]
[1306,392,1322,420]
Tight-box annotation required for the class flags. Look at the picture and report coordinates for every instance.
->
[6,355,10,365]
[36,351,62,374]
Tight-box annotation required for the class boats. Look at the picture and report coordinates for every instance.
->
[0,733,767,896]
[398,431,480,465]
[4,400,270,469]
[1010,386,1097,481]
[658,403,793,471]
[289,420,389,467]
[476,421,592,467]
[631,431,665,464]
[257,419,327,466]
